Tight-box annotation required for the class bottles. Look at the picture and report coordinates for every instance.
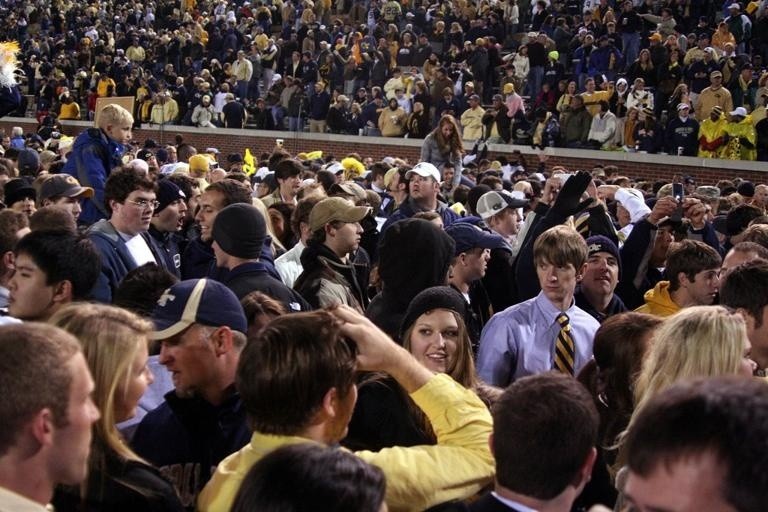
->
[661,110,668,129]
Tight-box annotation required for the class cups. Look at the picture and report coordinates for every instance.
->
[359,129,364,136]
[276,139,284,150]
[678,147,684,157]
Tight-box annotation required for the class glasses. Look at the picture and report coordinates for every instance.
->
[124,199,161,211]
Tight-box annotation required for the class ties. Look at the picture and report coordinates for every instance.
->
[553,313,576,379]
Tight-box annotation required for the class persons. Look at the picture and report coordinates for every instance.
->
[0,0,767,510]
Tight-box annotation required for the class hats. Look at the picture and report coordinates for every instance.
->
[307,198,373,232]
[402,285,466,322]
[213,202,265,259]
[155,181,185,212]
[145,278,249,340]
[583,234,622,281]
[443,186,529,253]
[2,147,95,208]
[405,162,442,185]
[190,156,210,170]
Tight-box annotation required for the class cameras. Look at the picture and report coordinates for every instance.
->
[276,139,284,148]
[672,182,683,200]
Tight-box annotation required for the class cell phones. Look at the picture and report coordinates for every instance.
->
[553,173,576,190]
